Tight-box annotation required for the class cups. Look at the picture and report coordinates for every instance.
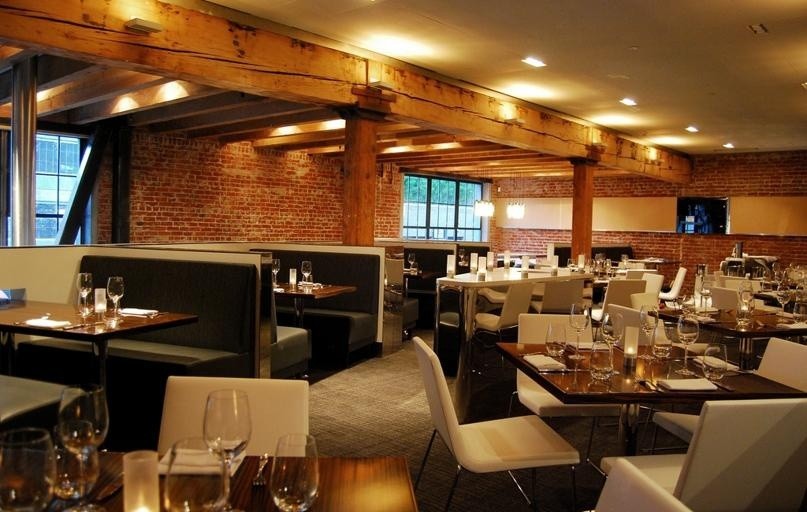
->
[446,254,457,279]
[77,288,92,315]
[520,255,529,278]
[269,432,319,512]
[487,251,495,272]
[549,256,560,278]
[503,250,511,271]
[476,256,487,282]
[94,288,107,321]
[302,275,314,291]
[578,254,586,274]
[469,252,478,275]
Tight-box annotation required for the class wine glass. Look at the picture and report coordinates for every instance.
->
[408,253,415,270]
[301,261,313,285]
[76,272,93,318]
[106,276,124,321]
[271,259,281,287]
[0,384,252,512]
[545,253,807,375]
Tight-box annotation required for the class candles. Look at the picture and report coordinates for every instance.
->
[94,288,106,322]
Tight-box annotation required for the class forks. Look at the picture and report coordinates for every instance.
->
[252,452,270,486]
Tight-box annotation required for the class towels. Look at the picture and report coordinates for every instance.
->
[274,288,284,292]
[118,307,159,319]
[25,319,71,329]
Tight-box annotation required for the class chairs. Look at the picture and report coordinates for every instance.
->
[158,375,309,457]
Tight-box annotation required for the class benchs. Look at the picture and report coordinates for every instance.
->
[0,374,77,437]
[92,245,311,381]
[17,255,255,450]
[249,249,381,368]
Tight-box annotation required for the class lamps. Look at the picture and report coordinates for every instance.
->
[507,172,526,219]
[0,301,199,451]
[474,168,494,217]
